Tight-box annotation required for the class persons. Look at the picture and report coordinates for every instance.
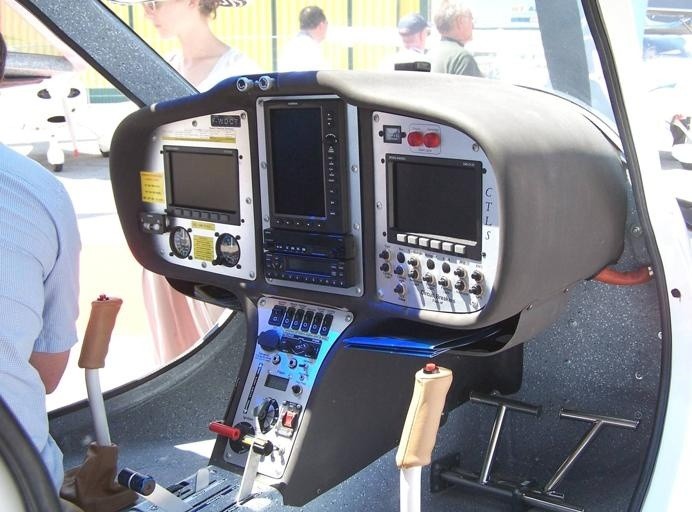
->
[0,32,81,512]
[424,1,484,78]
[111,0,262,95]
[669,113,692,170]
[381,13,434,72]
[278,5,331,70]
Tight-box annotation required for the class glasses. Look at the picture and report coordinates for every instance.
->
[141,0,164,11]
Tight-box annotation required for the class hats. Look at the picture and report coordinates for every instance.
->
[397,11,430,36]
